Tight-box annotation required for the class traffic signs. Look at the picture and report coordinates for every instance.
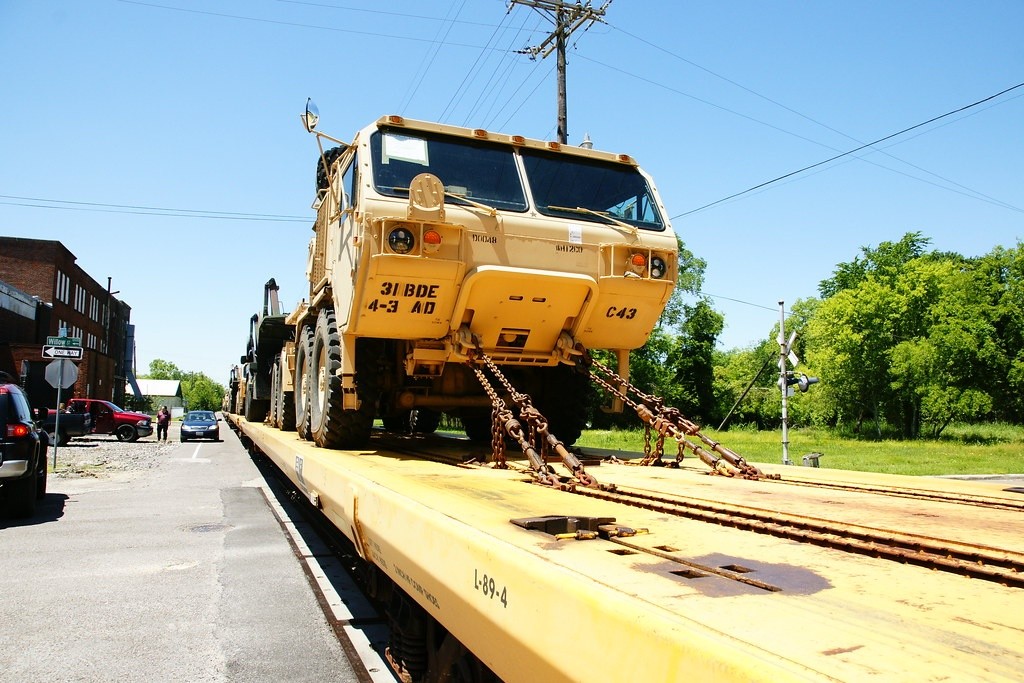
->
[42,345,83,360]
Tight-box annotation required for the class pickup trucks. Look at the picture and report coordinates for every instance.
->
[33,398,153,443]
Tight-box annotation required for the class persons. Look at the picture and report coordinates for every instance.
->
[156,404,170,442]
[205,414,211,419]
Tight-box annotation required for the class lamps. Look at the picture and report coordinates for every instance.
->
[98,380,101,385]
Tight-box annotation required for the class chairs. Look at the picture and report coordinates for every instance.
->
[191,416,196,420]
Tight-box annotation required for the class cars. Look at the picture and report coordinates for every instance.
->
[178,410,222,442]
[0,381,51,518]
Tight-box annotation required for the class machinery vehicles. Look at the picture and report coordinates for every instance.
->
[220,99,680,451]
